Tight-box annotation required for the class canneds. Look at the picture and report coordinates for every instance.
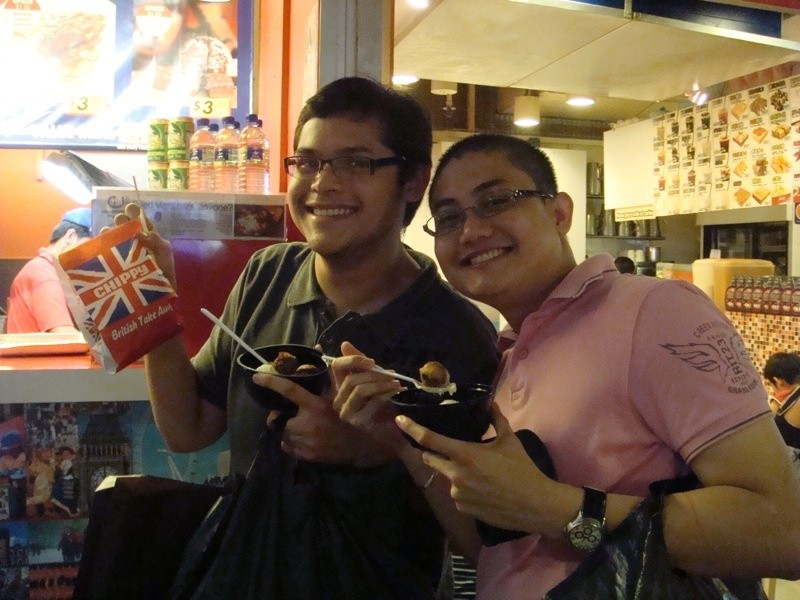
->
[148,115,195,191]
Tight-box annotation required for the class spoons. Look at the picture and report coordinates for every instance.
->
[320,354,455,393]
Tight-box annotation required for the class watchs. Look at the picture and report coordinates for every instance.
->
[562,484,607,552]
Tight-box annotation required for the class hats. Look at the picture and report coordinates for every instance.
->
[62,208,92,229]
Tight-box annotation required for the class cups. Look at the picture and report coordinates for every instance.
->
[657,110,729,190]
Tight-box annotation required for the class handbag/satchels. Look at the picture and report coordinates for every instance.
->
[542,472,768,600]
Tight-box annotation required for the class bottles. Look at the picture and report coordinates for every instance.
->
[725,274,800,317]
[189,114,270,194]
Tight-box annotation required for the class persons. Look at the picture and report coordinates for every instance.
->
[5,206,94,335]
[27,446,77,517]
[331,136,800,600]
[100,77,502,600]
[763,347,800,450]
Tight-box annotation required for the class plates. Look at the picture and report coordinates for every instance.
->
[0,332,88,356]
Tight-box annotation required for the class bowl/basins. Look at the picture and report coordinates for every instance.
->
[391,384,490,449]
[236,345,330,408]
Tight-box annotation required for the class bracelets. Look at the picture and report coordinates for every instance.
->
[419,471,439,490]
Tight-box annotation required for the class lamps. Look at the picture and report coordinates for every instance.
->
[565,95,595,106]
[514,95,540,127]
[684,89,707,106]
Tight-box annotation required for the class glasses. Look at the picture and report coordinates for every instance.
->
[284,154,406,178]
[423,188,555,237]
